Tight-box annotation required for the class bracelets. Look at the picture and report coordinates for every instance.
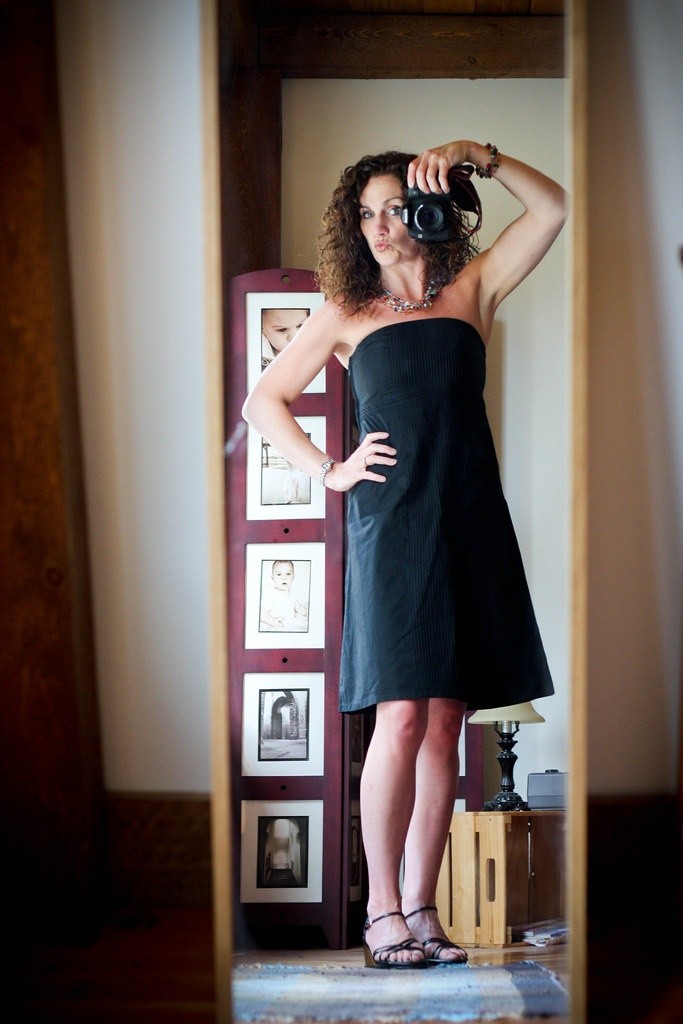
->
[476,143,501,178]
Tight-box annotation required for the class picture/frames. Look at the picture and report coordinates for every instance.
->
[246,416,327,520]
[244,542,326,650]
[241,672,325,777]
[246,292,326,394]
[239,799,323,903]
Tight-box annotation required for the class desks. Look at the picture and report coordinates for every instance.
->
[434,811,566,950]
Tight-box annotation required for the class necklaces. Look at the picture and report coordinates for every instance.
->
[379,282,443,312]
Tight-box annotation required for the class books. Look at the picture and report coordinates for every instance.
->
[522,927,570,947]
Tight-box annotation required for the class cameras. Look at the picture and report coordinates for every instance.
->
[401,183,455,244]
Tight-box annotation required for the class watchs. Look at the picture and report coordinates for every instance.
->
[320,459,335,485]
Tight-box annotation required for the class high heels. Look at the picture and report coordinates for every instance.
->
[405,906,468,967]
[362,912,427,969]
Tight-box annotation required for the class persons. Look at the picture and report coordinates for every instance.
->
[260,561,308,628]
[261,310,307,360]
[280,699,293,739]
[241,138,570,970]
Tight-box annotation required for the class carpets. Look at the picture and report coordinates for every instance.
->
[230,961,569,1023]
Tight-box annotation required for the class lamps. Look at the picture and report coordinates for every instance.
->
[467,702,546,810]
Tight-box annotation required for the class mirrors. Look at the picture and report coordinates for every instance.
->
[213,0,571,1024]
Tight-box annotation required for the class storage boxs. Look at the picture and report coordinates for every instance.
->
[527,770,567,810]
